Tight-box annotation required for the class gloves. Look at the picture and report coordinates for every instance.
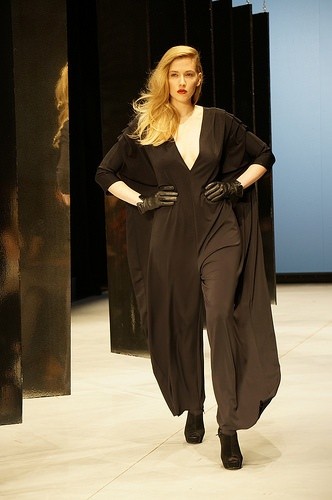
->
[204,179,243,202]
[136,185,178,215]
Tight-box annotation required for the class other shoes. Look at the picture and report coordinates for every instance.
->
[185,409,205,444]
[216,427,243,470]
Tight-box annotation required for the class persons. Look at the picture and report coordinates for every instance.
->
[95,45,281,469]
